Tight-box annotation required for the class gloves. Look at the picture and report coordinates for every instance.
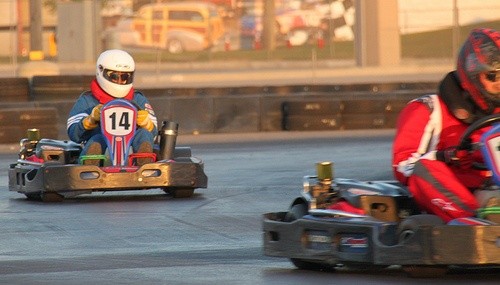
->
[438,145,472,169]
[136,109,155,131]
[82,103,105,129]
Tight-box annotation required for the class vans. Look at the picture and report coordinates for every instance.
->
[119,2,225,54]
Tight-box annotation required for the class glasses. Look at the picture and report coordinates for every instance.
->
[486,70,500,82]
[109,72,129,80]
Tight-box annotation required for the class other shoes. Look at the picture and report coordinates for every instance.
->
[483,196,500,224]
[136,141,152,165]
[84,141,103,164]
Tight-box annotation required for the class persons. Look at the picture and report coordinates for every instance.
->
[67,49,158,165]
[393,28,500,223]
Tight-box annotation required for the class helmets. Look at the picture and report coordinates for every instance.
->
[458,26,500,112]
[96,49,136,98]
